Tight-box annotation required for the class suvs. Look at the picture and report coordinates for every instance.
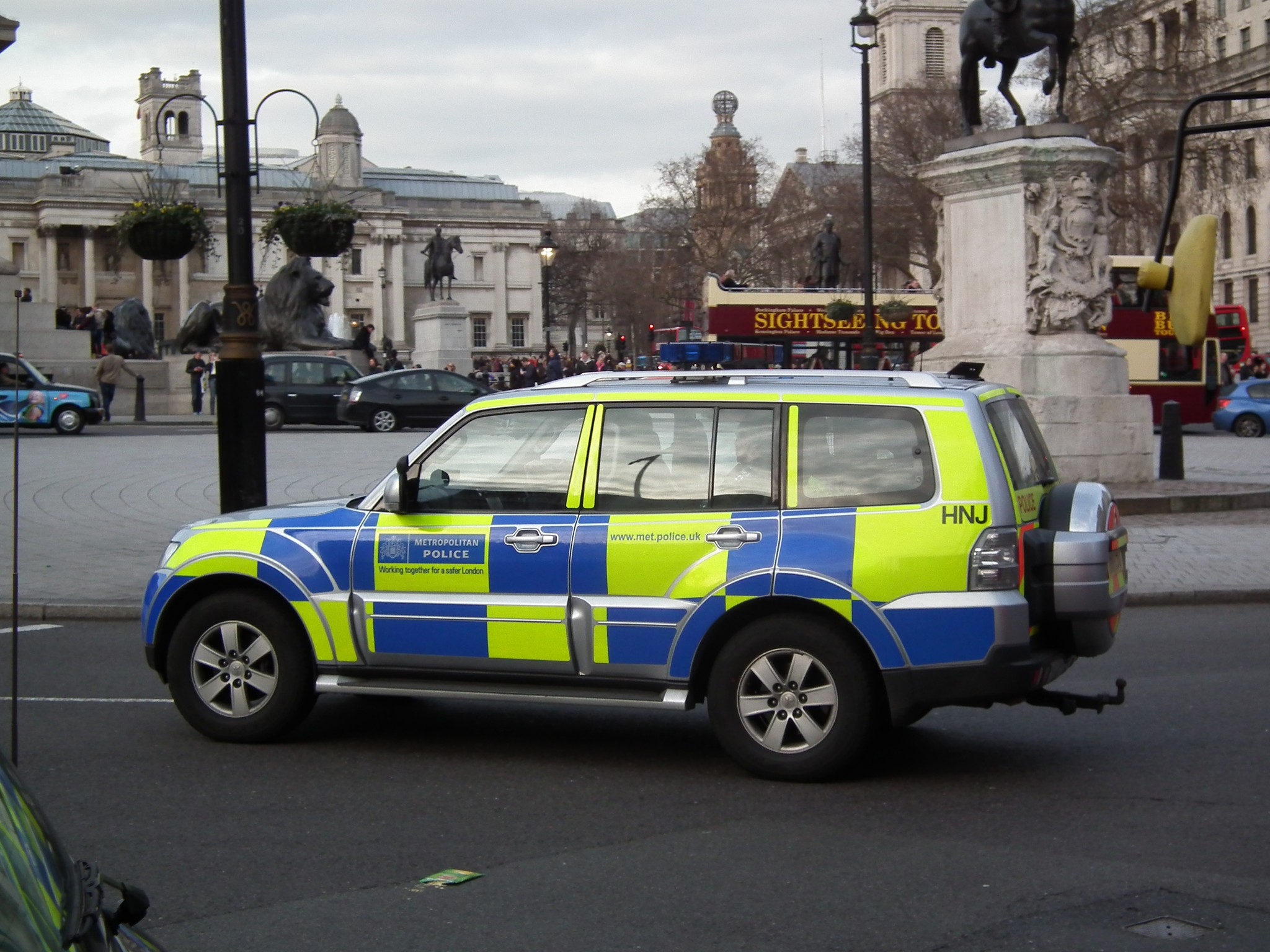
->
[261,354,367,430]
[139,341,1129,785]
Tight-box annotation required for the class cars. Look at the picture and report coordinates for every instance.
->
[1212,378,1270,439]
[0,352,106,435]
[337,368,507,432]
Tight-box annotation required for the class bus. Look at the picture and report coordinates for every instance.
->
[694,254,1254,433]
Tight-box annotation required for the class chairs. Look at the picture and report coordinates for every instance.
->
[662,414,712,492]
[311,362,322,383]
[295,362,309,383]
[610,420,666,495]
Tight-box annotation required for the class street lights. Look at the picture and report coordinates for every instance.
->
[850,0,885,372]
[534,227,561,367]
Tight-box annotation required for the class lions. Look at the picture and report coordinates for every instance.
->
[173,256,376,352]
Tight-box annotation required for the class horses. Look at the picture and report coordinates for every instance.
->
[958,1,1076,136]
[428,235,463,301]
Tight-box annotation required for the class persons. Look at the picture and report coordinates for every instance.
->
[1221,353,1232,384]
[604,411,670,493]
[792,275,817,292]
[1037,209,1073,281]
[1084,214,1113,282]
[25,391,45,422]
[21,288,32,301]
[795,346,833,369]
[186,350,220,415]
[1240,358,1267,380]
[55,306,115,358]
[812,218,844,294]
[421,228,457,280]
[906,280,918,293]
[986,0,1008,60]
[328,324,456,384]
[0,363,26,386]
[96,344,139,421]
[722,270,748,292]
[715,416,773,508]
[469,347,680,391]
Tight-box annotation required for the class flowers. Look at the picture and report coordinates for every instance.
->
[106,156,223,283]
[252,152,381,285]
[820,285,862,327]
[877,286,918,322]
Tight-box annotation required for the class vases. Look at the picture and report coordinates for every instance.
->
[826,310,855,321]
[270,216,358,259]
[881,310,913,322]
[126,225,197,264]
